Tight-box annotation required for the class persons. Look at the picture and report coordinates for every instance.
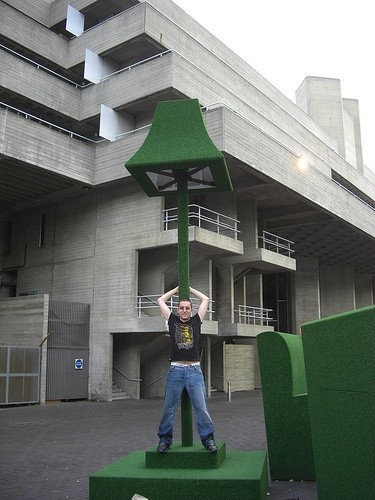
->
[157,286,219,452]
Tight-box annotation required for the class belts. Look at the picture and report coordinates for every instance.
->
[171,362,200,367]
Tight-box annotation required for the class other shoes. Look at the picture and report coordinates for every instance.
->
[157,444,170,452]
[208,442,218,452]
